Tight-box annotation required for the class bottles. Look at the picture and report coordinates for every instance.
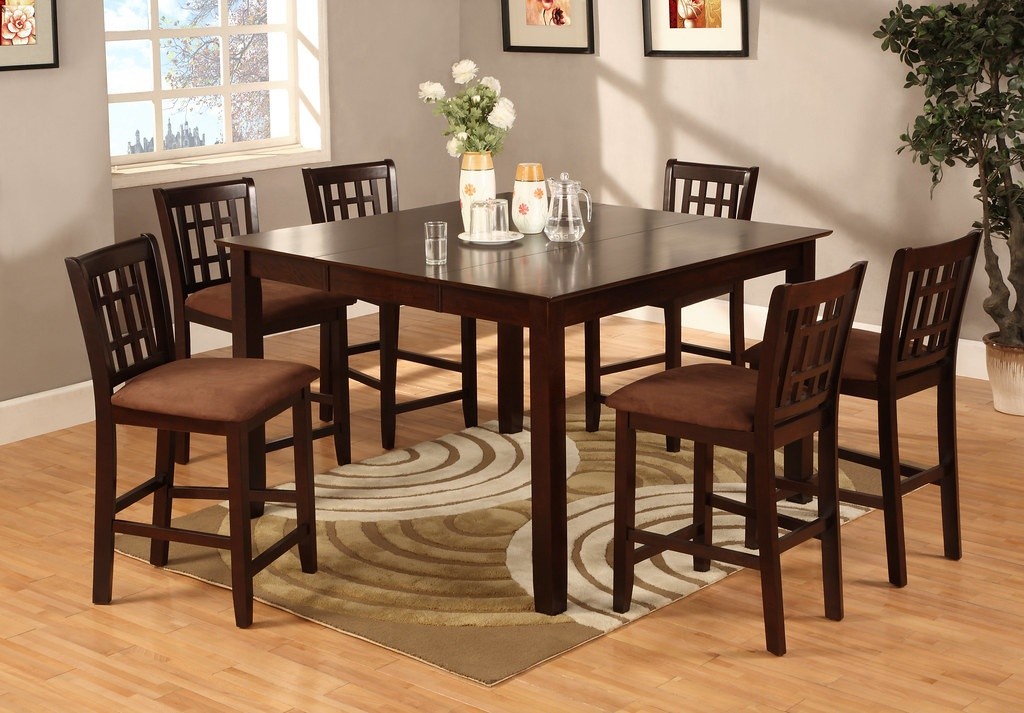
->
[511,163,549,234]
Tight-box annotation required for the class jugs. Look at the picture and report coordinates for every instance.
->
[543,172,592,243]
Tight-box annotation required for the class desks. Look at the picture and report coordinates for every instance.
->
[215,190,834,615]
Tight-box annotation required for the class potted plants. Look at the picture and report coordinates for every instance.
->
[872,1,1024,416]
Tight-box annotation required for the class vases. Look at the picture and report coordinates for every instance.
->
[512,163,548,234]
[460,150,496,232]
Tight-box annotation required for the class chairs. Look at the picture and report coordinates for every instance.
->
[605,259,870,657]
[304,158,478,450]
[739,228,982,588]
[153,177,358,470]
[582,158,759,454]
[61,232,321,629]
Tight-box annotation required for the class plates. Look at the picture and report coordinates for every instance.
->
[458,231,524,245]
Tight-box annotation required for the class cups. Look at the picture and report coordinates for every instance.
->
[489,199,509,239]
[470,201,492,241]
[424,221,448,265]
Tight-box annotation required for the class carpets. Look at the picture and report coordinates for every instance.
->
[116,383,932,687]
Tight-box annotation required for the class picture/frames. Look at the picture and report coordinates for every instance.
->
[0,0,59,71]
[641,0,750,58]
[501,0,594,54]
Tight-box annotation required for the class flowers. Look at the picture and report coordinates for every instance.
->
[419,58,518,159]
